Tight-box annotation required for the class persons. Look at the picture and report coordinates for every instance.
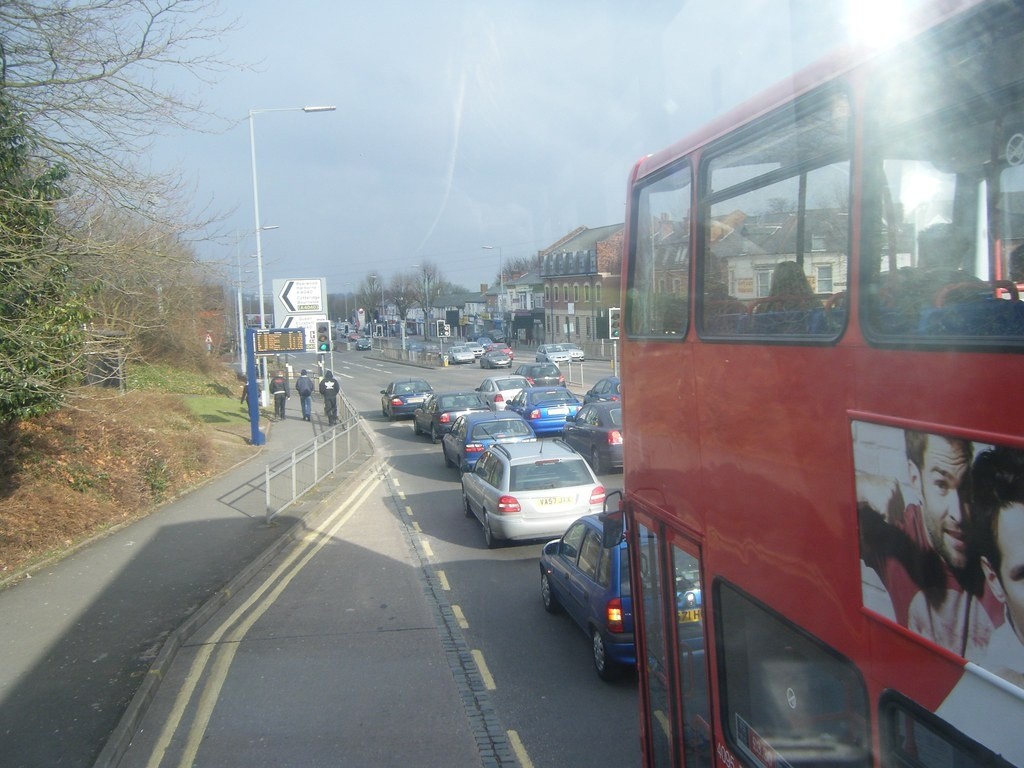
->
[761,261,824,311]
[295,370,314,421]
[269,371,291,421]
[900,221,972,337]
[319,371,339,425]
[856,427,1024,689]
[240,384,261,418]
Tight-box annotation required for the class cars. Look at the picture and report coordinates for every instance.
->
[540,511,702,680]
[379,378,440,420]
[477,338,494,349]
[447,346,476,364]
[399,340,442,360]
[485,345,515,359]
[412,391,492,444]
[338,323,361,343]
[505,386,583,439]
[474,374,533,412]
[355,339,371,350]
[443,411,547,472]
[509,362,568,389]
[535,344,572,366]
[585,375,621,403]
[562,400,627,470]
[559,343,585,361]
[463,342,485,358]
[479,351,513,369]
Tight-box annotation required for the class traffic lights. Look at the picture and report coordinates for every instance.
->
[607,307,620,341]
[315,320,333,354]
[437,320,454,339]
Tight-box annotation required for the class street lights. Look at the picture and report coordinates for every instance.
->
[346,283,357,314]
[412,264,431,341]
[237,224,281,377]
[481,245,507,307]
[371,275,385,325]
[247,103,337,410]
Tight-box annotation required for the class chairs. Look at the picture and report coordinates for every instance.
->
[660,280,1024,335]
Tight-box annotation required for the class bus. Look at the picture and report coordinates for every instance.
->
[614,0,1022,766]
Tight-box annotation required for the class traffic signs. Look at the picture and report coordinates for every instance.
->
[273,278,330,354]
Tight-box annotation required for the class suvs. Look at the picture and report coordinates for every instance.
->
[458,438,611,549]
